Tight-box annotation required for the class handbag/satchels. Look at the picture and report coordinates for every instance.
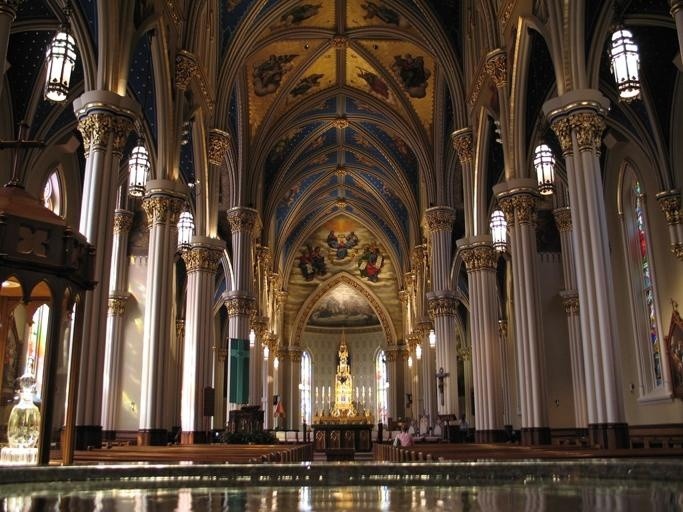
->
[393,438,400,447]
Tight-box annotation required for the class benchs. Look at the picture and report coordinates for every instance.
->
[73,440,313,469]
[369,439,683,464]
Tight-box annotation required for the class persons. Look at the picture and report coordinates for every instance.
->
[248,1,431,103]
[294,230,385,284]
[392,422,415,447]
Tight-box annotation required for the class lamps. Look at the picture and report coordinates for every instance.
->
[44,6,75,105]
[128,98,153,198]
[533,120,556,197]
[488,196,509,255]
[176,191,195,253]
[611,8,644,104]
